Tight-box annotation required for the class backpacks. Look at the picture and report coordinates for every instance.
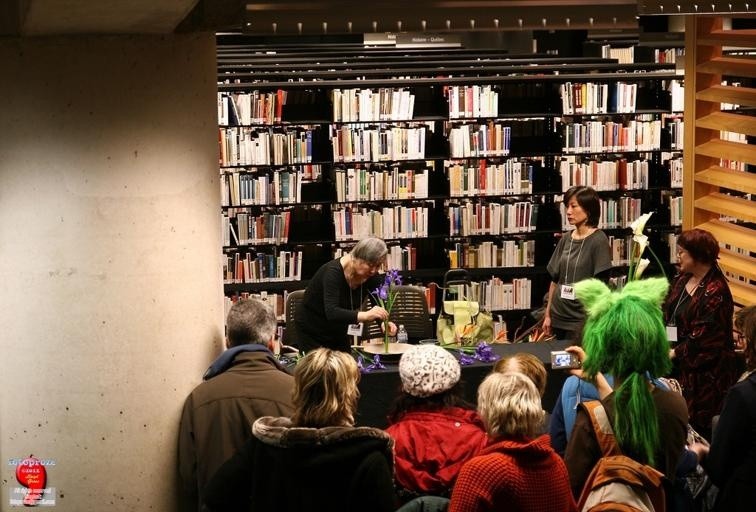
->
[577,400,667,512]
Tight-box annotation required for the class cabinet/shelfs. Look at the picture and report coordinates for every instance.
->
[218,46,685,328]
[686,16,752,326]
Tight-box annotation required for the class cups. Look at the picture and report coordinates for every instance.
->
[419,339,441,345]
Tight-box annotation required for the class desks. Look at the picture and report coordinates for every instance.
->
[281,338,580,426]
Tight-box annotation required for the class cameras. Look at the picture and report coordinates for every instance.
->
[550,351,580,370]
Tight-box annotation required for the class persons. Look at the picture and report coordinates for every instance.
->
[494,352,551,429]
[702,305,755,512]
[550,371,668,455]
[178,296,401,512]
[563,277,689,512]
[383,343,487,494]
[542,184,613,340]
[446,371,581,512]
[293,234,388,360]
[662,228,735,444]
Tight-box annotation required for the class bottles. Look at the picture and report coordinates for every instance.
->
[396,324,408,344]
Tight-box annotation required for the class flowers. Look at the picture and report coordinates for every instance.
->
[455,342,497,364]
[353,353,384,373]
[370,267,401,350]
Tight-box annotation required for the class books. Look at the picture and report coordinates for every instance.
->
[216,88,323,353]
[329,87,430,273]
[440,85,539,312]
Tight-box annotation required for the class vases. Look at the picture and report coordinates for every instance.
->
[352,342,412,363]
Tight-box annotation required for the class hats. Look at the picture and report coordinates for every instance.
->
[399,344,461,398]
